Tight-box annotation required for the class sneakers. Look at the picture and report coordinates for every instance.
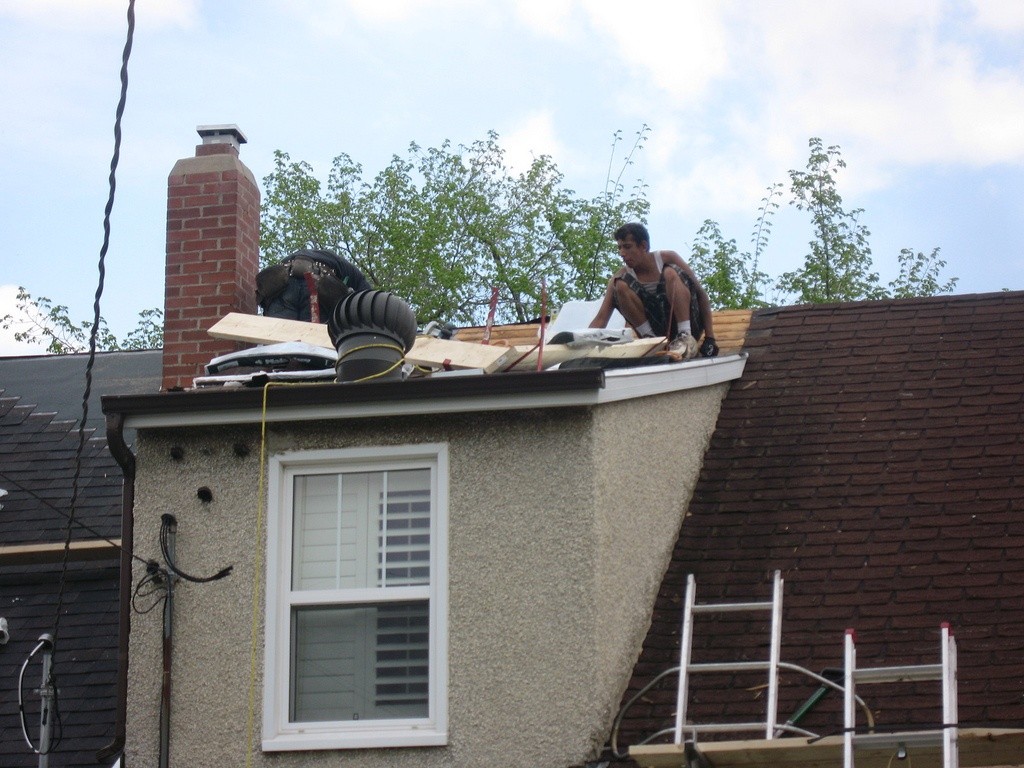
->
[667,330,695,362]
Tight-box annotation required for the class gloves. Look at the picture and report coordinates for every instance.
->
[699,336,719,358]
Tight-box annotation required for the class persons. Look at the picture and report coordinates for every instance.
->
[588,223,720,358]
[256,249,371,324]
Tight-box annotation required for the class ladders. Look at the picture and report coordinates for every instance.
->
[843,620,959,768]
[675,568,784,745]
